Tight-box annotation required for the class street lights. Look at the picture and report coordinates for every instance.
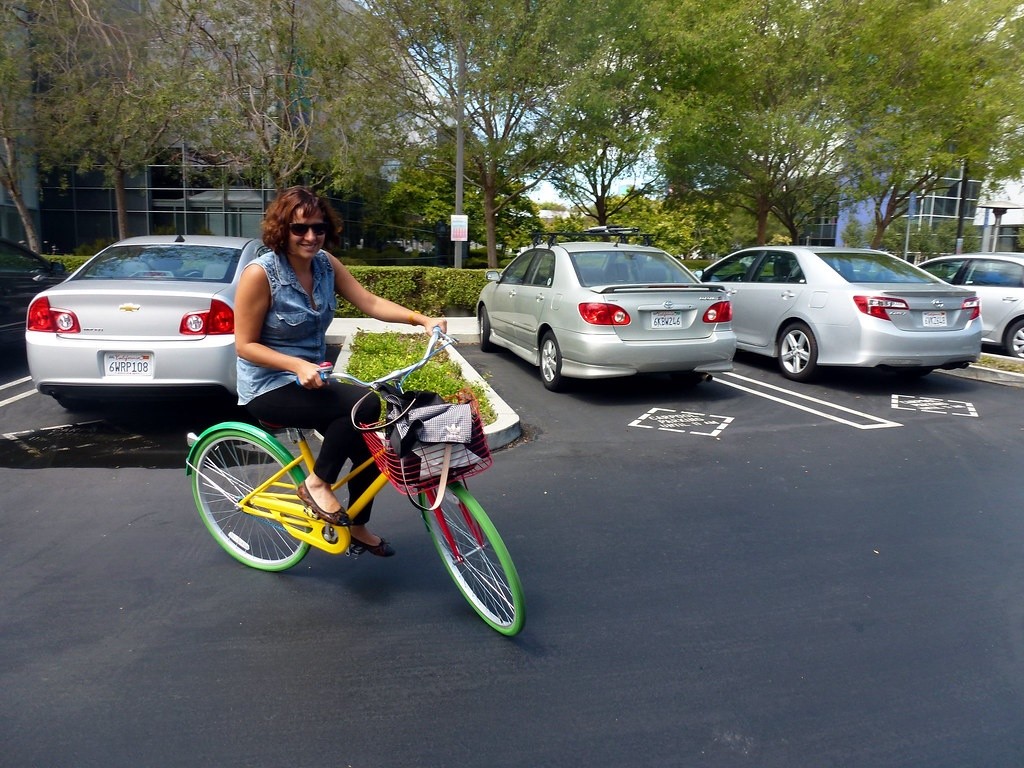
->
[976,199,1024,253]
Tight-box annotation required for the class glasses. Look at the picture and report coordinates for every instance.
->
[288,222,329,236]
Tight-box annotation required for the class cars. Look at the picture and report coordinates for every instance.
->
[0,238,69,343]
[476,225,738,394]
[18,238,74,256]
[914,252,1024,359]
[25,235,271,430]
[693,245,982,384]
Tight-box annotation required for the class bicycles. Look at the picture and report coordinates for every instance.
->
[185,326,525,640]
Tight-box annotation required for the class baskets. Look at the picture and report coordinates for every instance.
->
[359,386,494,496]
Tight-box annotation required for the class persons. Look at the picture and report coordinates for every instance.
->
[233,188,448,557]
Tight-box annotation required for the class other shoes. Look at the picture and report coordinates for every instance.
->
[328,524,395,558]
[296,480,353,527]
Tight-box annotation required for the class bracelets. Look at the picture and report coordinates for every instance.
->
[408,310,421,326]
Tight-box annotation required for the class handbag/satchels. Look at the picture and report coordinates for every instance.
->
[350,382,489,511]
